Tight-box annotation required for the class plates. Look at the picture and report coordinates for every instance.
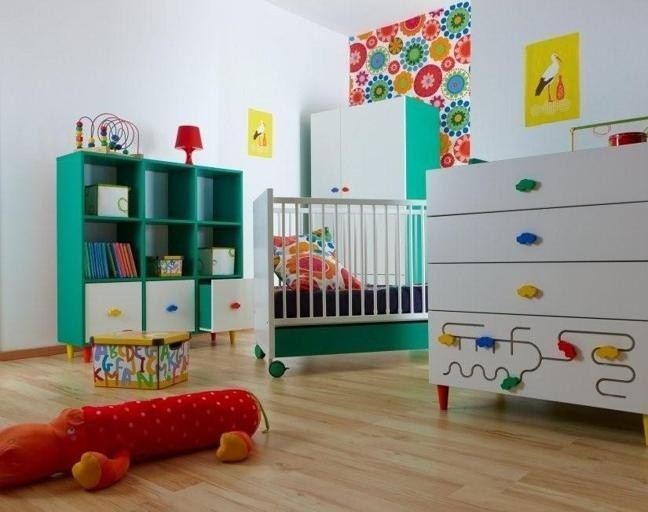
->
[56,150,254,360]
[425,139,648,444]
[309,97,440,288]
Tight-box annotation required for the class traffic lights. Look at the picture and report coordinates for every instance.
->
[272,228,363,290]
[0,389,273,493]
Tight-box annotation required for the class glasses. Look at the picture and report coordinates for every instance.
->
[175,124,205,164]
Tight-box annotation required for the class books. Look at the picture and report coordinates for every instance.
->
[91,331,190,389]
[147,254,182,276]
[85,184,130,216]
[199,246,236,275]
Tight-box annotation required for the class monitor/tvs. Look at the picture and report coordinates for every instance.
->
[82,239,139,280]
